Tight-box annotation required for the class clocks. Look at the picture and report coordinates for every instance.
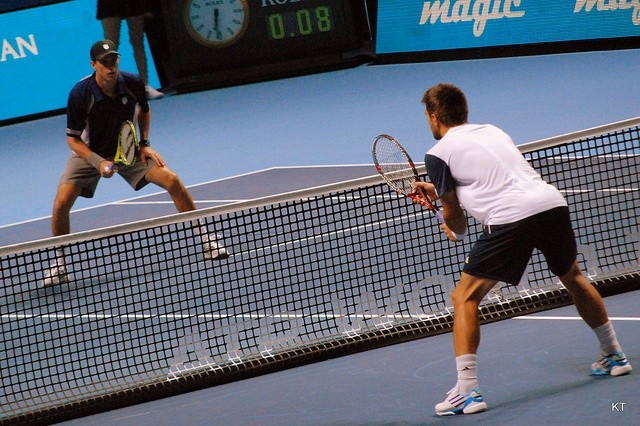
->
[184,0,250,48]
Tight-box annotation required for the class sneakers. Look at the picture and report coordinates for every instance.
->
[203,235,229,258]
[590,351,632,375]
[41,262,71,285]
[434,383,488,416]
[145,83,164,99]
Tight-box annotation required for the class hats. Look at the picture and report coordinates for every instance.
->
[90,39,121,61]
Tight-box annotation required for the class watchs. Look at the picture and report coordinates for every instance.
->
[138,140,151,146]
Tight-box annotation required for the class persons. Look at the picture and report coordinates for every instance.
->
[41,40,230,288]
[413,83,632,416]
[95,0,164,100]
